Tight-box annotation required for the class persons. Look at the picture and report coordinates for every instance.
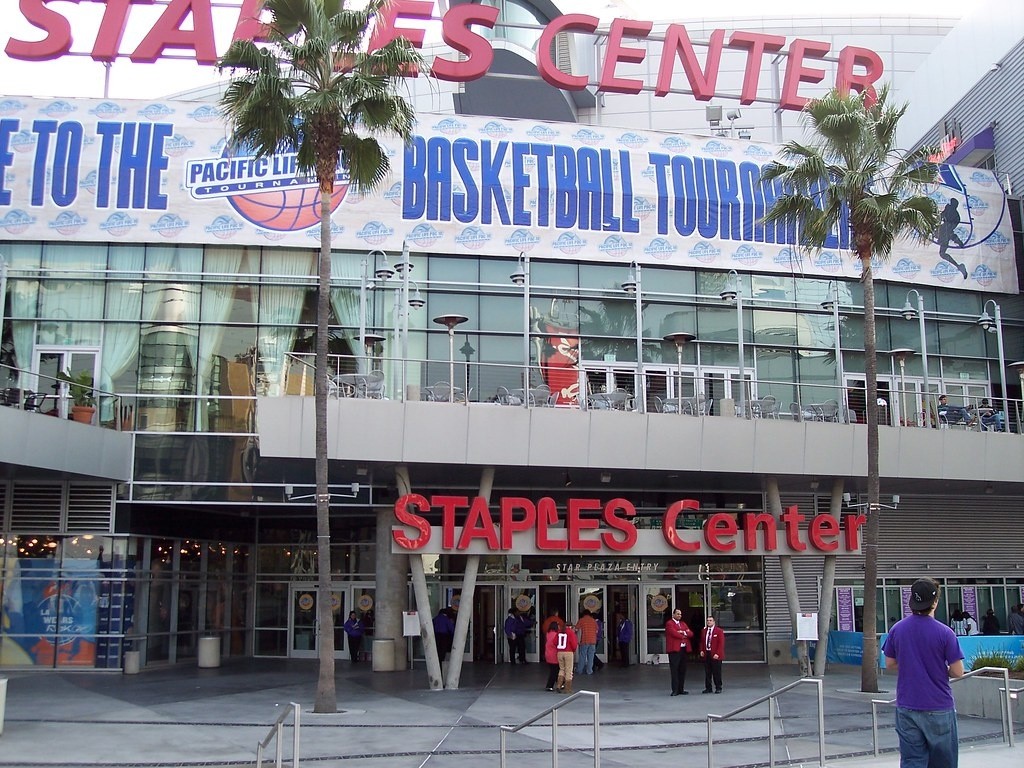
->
[617,615,633,667]
[432,599,463,689]
[507,607,538,664]
[178,607,186,623]
[951,603,1024,636]
[665,608,694,696]
[699,616,725,693]
[365,610,375,636]
[881,576,967,768]
[938,395,975,426]
[344,611,364,664]
[978,398,1002,432]
[691,608,704,650]
[542,607,602,693]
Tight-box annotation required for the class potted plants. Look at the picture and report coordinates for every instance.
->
[57,367,99,425]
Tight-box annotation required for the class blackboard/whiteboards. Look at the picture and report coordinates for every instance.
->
[796,612,819,640]
[403,611,421,636]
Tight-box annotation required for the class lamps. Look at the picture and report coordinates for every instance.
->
[565,469,571,488]
[601,470,611,482]
[706,105,755,140]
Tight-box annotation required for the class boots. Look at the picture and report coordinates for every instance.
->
[557,675,565,693]
[564,679,572,694]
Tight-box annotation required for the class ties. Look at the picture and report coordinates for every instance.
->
[707,629,711,649]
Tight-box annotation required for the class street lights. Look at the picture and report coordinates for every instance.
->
[719,269,746,418]
[900,289,931,429]
[820,280,845,424]
[622,260,642,410]
[433,314,468,403]
[509,251,530,407]
[977,300,1009,432]
[886,348,917,427]
[663,332,697,414]
[1007,361,1024,410]
[393,241,414,401]
[357,248,395,373]
[399,281,425,401]
[353,333,385,374]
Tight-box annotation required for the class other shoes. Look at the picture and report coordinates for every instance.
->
[715,685,722,693]
[703,688,713,693]
[679,691,689,695]
[545,687,556,691]
[671,691,680,696]
[522,661,530,664]
[597,663,604,671]
[560,685,564,688]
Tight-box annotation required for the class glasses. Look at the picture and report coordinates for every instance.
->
[706,620,713,622]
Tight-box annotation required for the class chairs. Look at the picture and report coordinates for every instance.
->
[849,409,857,423]
[324,367,384,399]
[916,406,997,429]
[419,380,840,423]
[0,387,47,414]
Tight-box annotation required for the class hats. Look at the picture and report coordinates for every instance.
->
[909,576,938,611]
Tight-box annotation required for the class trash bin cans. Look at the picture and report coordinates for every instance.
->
[372,638,395,672]
[198,634,221,668]
[125,651,139,674]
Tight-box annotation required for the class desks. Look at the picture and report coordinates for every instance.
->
[588,393,633,410]
[970,408,993,432]
[736,400,775,419]
[508,388,550,408]
[800,404,835,423]
[419,386,463,401]
[337,373,379,398]
